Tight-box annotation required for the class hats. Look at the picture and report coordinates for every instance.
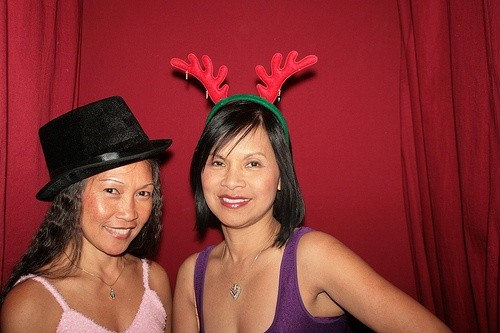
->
[34,94,174,202]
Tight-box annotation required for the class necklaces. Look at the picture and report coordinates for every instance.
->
[62,249,128,300]
[220,221,280,301]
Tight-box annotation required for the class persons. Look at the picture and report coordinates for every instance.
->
[169,50,453,333]
[0,95,174,333]
